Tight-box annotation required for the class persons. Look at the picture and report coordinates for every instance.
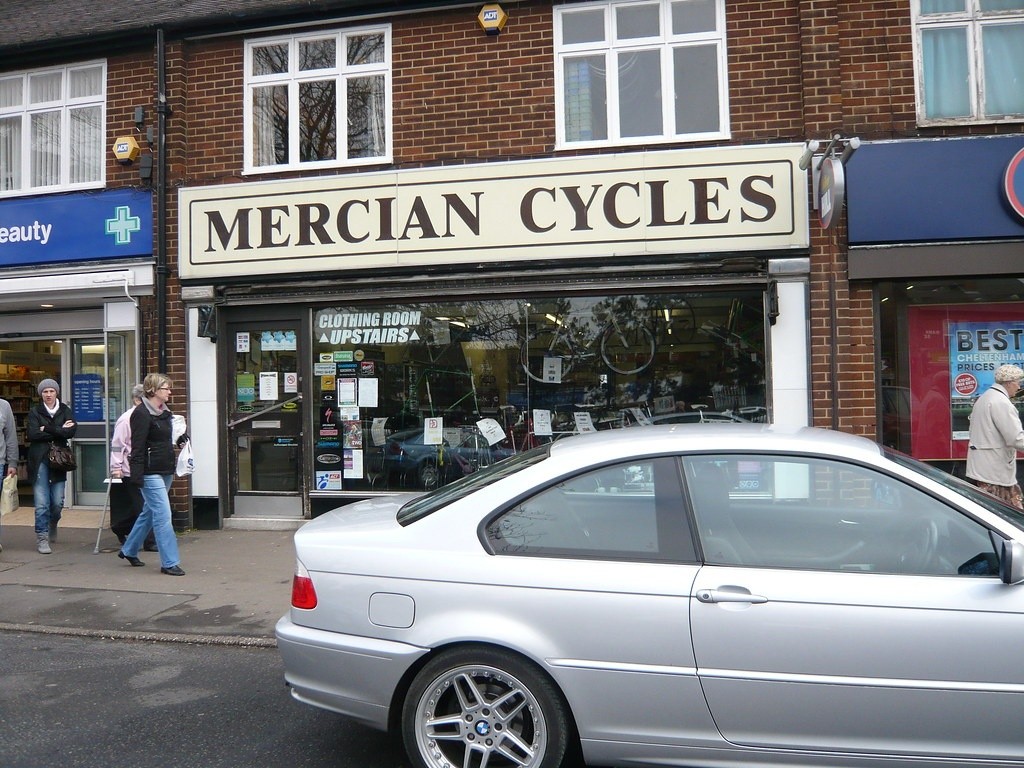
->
[27,378,78,554]
[966,364,1024,511]
[0,399,19,553]
[110,384,158,552]
[697,465,766,567]
[118,373,189,577]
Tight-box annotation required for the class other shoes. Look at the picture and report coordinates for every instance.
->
[117,534,127,544]
[145,545,159,551]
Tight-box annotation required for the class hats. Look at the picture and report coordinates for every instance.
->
[994,365,1024,382]
[37,378,59,397]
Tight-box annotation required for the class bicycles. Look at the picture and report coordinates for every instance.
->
[454,404,646,474]
[519,294,655,385]
[642,293,698,347]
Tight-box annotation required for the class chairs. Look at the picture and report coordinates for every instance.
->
[692,463,759,564]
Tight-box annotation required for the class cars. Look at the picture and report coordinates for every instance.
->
[274,423,1024,766]
[376,426,521,490]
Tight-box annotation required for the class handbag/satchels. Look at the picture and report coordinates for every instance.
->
[48,439,78,471]
[176,438,193,477]
[0,474,19,516]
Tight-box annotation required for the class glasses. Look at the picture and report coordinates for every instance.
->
[157,387,169,391]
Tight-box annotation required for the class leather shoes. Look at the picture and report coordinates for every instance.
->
[118,549,145,567]
[160,564,185,576]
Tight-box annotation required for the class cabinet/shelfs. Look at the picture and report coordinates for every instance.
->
[0,378,38,482]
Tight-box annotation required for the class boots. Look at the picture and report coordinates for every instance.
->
[48,522,58,541]
[36,531,52,553]
